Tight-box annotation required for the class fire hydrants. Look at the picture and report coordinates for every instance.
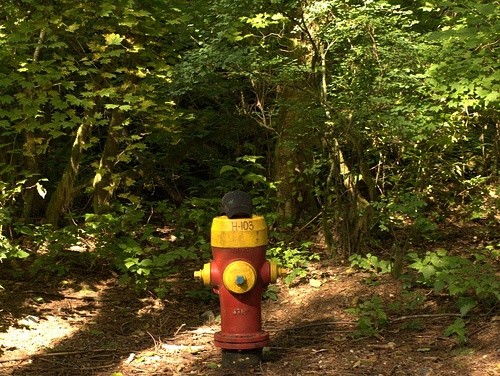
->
[193,190,293,369]
